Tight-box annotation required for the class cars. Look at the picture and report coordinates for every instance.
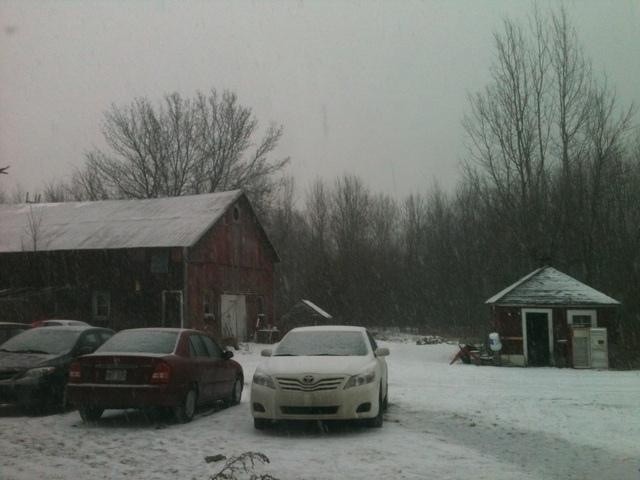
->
[0,319,245,424]
[250,325,390,429]
